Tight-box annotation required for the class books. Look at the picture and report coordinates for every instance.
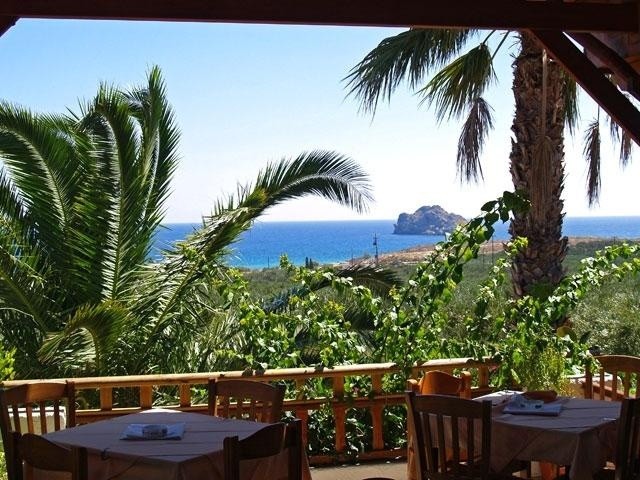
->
[121,422,184,441]
[503,395,562,416]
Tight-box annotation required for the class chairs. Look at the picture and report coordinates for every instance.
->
[407,369,472,400]
[223,419,303,479]
[8,429,90,480]
[584,353,640,403]
[206,377,286,423]
[401,390,532,480]
[557,395,640,480]
[0,380,77,477]
